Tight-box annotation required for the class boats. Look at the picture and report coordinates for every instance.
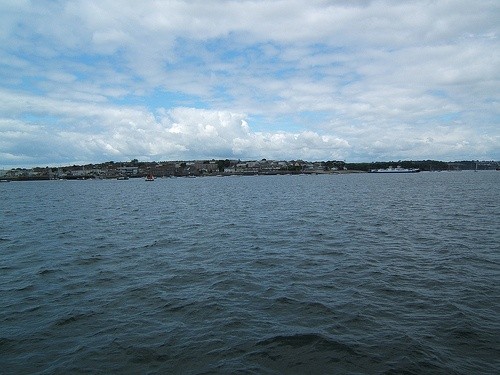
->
[368,166,421,173]
[117,177,129,180]
[145,174,154,181]
